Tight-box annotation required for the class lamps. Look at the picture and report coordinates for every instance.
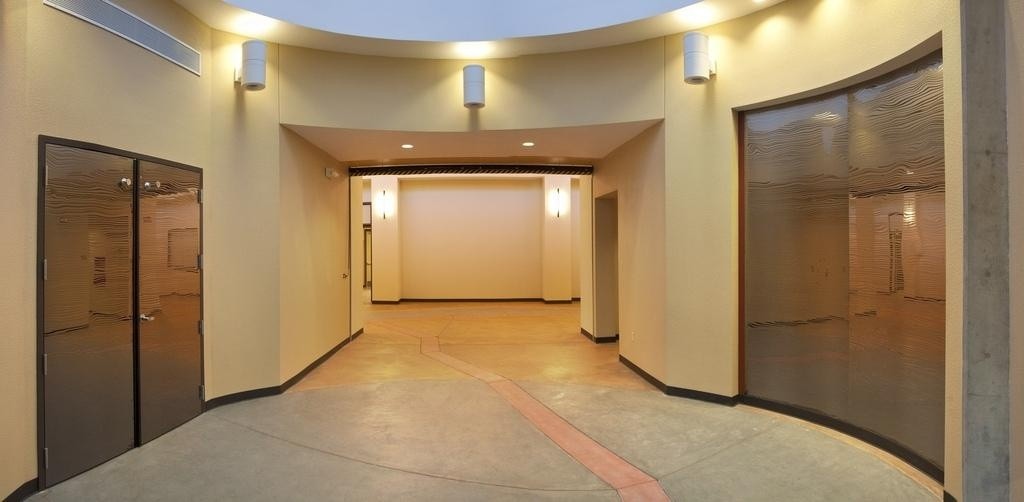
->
[683,32,717,84]
[462,65,485,109]
[234,39,266,90]
